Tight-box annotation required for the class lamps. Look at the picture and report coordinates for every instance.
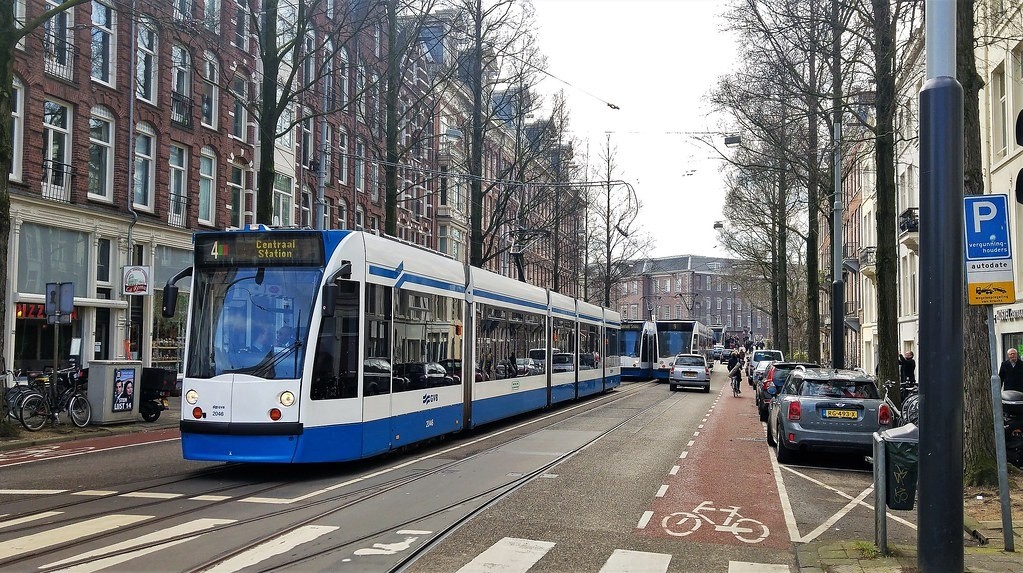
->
[725,134,742,148]
[714,222,723,230]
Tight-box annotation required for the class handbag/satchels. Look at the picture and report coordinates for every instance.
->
[728,362,741,378]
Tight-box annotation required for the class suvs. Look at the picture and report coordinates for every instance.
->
[755,361,822,422]
[768,364,892,470]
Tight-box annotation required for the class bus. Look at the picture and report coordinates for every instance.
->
[706,323,726,352]
[161,222,621,477]
[652,318,715,383]
[620,318,656,382]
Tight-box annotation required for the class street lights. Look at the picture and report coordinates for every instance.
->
[724,135,790,359]
[384,128,463,236]
[713,223,780,350]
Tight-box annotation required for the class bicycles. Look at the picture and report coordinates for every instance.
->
[0,358,178,432]
[882,379,920,427]
[728,374,739,398]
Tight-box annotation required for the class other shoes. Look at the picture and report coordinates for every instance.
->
[738,390,740,391]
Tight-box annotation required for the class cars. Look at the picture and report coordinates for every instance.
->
[714,344,784,390]
[668,351,711,393]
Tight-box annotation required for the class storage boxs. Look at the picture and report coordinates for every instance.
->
[144,366,177,390]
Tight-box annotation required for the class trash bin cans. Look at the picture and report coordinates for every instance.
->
[880,421,920,511]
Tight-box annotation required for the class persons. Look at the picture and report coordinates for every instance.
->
[898,351,915,387]
[727,349,745,393]
[251,327,269,357]
[744,338,765,354]
[114,379,133,410]
[998,348,1023,393]
[276,326,296,350]
[485,348,494,378]
[724,335,741,349]
[479,348,486,371]
[507,352,517,379]
